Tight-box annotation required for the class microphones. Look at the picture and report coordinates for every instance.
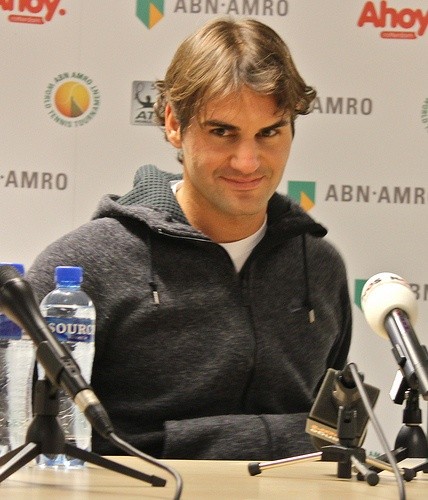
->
[0,265,114,438]
[305,363,380,450]
[361,272,428,401]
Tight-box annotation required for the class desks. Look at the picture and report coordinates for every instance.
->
[0,456,428,500]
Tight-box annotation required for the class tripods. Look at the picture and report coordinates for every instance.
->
[248,343,428,487]
[0,367,167,486]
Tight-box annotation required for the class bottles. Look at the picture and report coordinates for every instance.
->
[38,266,95,468]
[0,264,36,466]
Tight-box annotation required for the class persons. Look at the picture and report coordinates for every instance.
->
[27,16,352,460]
